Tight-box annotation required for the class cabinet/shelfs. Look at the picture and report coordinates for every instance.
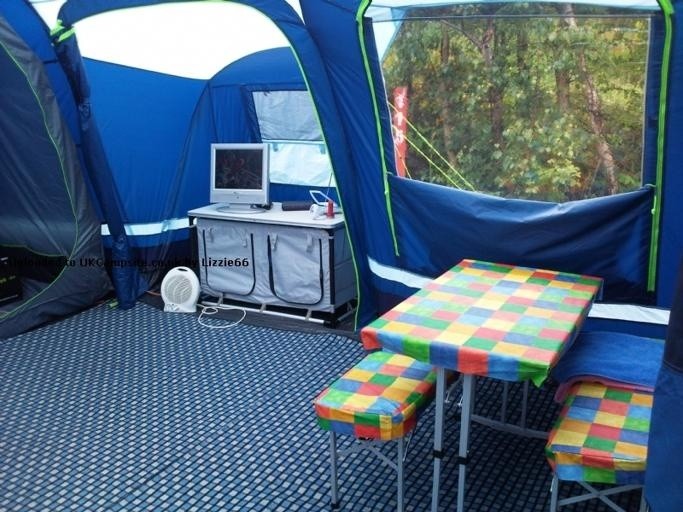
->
[186,201,358,328]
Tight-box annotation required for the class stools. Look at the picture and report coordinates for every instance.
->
[316,349,463,512]
[541,382,654,510]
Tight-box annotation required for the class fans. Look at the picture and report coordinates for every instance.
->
[159,264,200,315]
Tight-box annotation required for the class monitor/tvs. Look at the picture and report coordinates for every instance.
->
[210,143,270,214]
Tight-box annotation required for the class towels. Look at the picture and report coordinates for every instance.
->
[553,375,656,402]
[550,330,666,388]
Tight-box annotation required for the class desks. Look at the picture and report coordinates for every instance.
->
[359,253,609,511]
